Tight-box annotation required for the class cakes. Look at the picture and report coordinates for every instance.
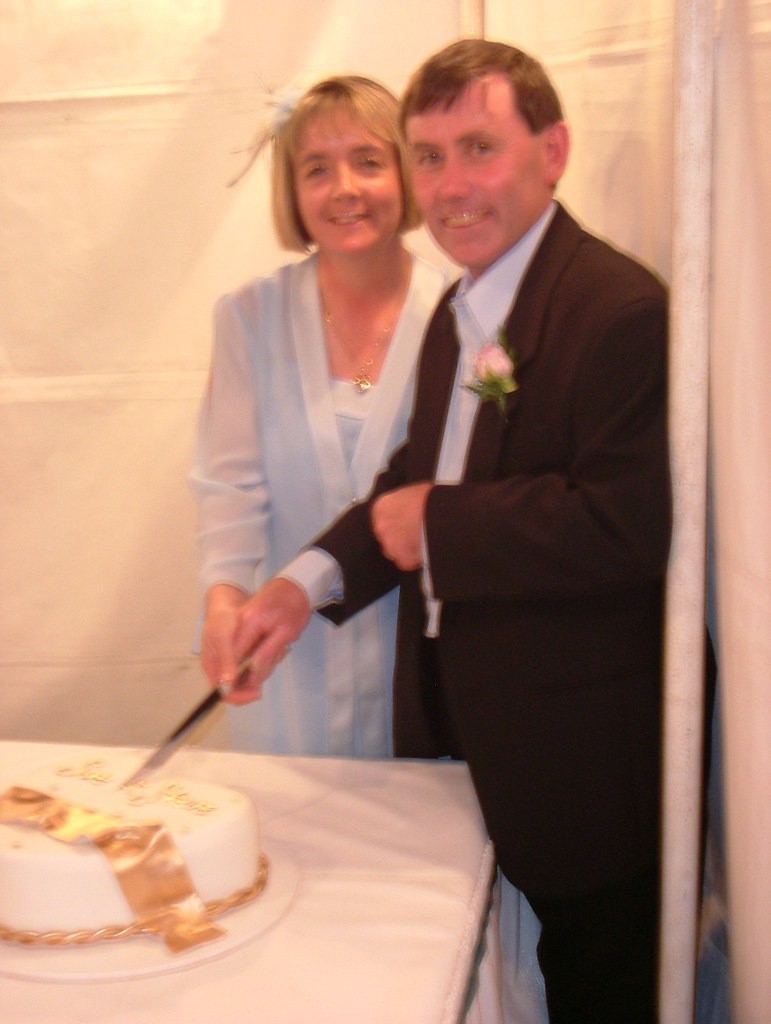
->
[0,759,260,933]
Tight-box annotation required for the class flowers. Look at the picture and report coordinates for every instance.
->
[463,329,519,418]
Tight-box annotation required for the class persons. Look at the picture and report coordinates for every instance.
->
[233,38,671,1024]
[189,74,550,1023]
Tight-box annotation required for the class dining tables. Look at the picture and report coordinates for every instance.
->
[0,743,550,1024]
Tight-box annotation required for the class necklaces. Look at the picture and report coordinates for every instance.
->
[322,298,391,393]
